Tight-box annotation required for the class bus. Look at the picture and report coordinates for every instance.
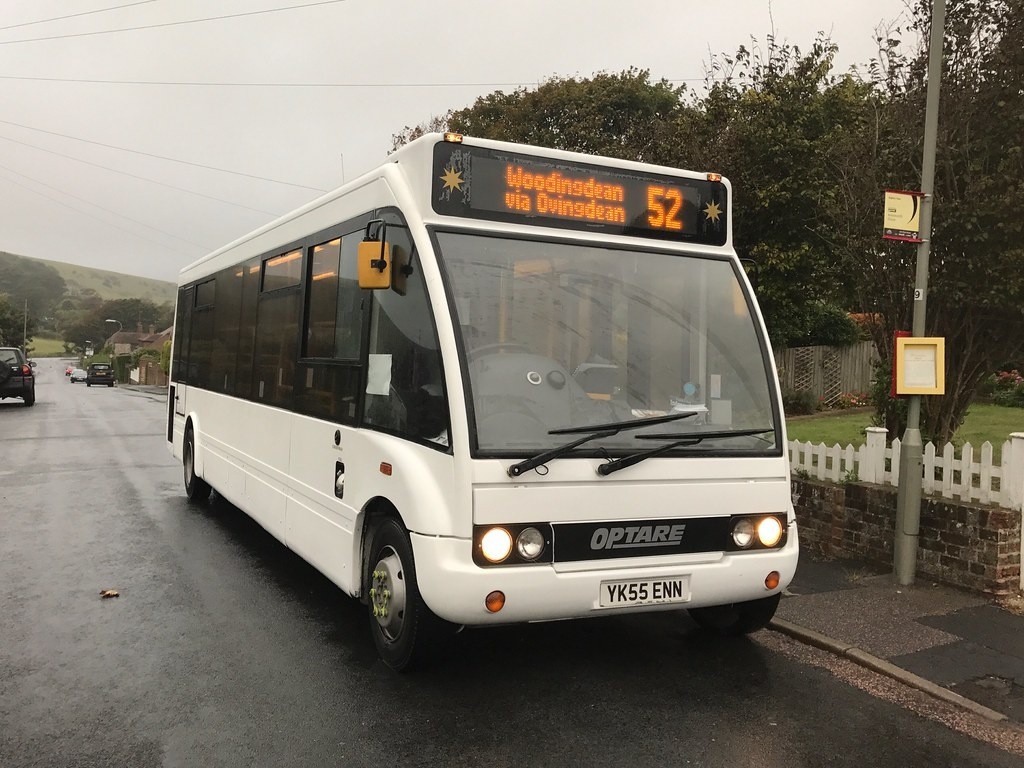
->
[163,130,803,673]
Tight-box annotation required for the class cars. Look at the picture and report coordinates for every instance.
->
[66,366,87,383]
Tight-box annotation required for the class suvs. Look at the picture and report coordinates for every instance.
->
[86,363,115,387]
[0,347,37,407]
